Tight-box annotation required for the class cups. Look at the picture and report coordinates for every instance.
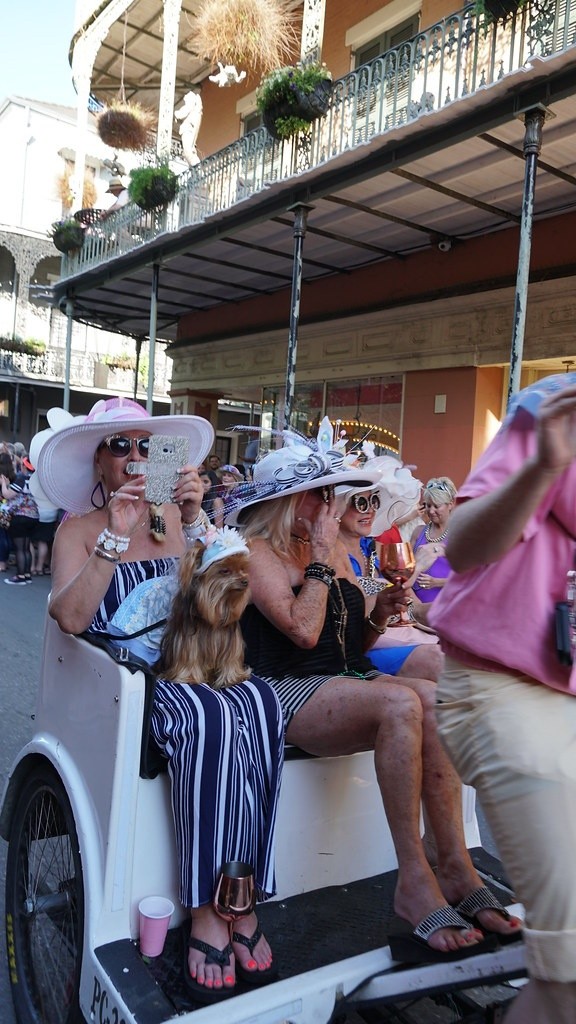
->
[138,896,175,957]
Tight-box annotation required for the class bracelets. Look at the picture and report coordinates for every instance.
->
[92,528,130,565]
[374,628,387,634]
[104,210,108,215]
[368,608,389,630]
[304,560,336,588]
[180,508,210,547]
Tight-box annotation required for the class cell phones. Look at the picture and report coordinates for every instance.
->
[145,435,189,504]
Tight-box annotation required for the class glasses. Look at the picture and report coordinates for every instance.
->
[99,434,150,458]
[314,485,335,503]
[426,481,454,501]
[352,489,381,513]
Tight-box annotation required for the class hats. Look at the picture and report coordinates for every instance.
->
[28,397,216,517]
[216,464,245,483]
[326,419,423,537]
[224,415,382,527]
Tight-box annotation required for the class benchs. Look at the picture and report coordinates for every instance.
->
[36,588,488,931]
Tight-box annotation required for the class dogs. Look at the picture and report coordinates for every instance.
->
[148,525,252,691]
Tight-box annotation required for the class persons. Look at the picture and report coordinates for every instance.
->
[39,396,284,992]
[427,370,576,1024]
[210,66,246,88]
[333,457,448,683]
[0,441,66,585]
[204,420,527,963]
[373,464,459,603]
[175,91,203,176]
[99,179,151,224]
[196,455,256,532]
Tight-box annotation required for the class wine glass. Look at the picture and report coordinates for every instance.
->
[212,862,256,946]
[380,542,418,627]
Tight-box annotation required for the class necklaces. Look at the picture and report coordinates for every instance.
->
[291,532,310,545]
[360,547,369,577]
[423,521,449,543]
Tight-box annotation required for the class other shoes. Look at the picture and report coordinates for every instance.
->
[23,574,32,584]
[29,568,51,577]
[3,575,27,586]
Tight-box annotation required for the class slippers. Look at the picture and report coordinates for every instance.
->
[179,915,238,999]
[445,885,526,945]
[229,917,280,984]
[389,903,496,965]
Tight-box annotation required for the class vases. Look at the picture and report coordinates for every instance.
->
[262,79,332,140]
[54,225,84,253]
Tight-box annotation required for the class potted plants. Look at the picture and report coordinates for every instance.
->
[472,0,532,41]
[94,353,150,393]
[128,164,180,211]
[23,339,45,358]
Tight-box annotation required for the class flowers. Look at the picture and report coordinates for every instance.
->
[47,219,83,241]
[217,526,247,550]
[255,58,333,140]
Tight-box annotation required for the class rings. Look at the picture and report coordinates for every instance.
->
[109,491,118,498]
[433,546,438,552]
[333,516,341,522]
